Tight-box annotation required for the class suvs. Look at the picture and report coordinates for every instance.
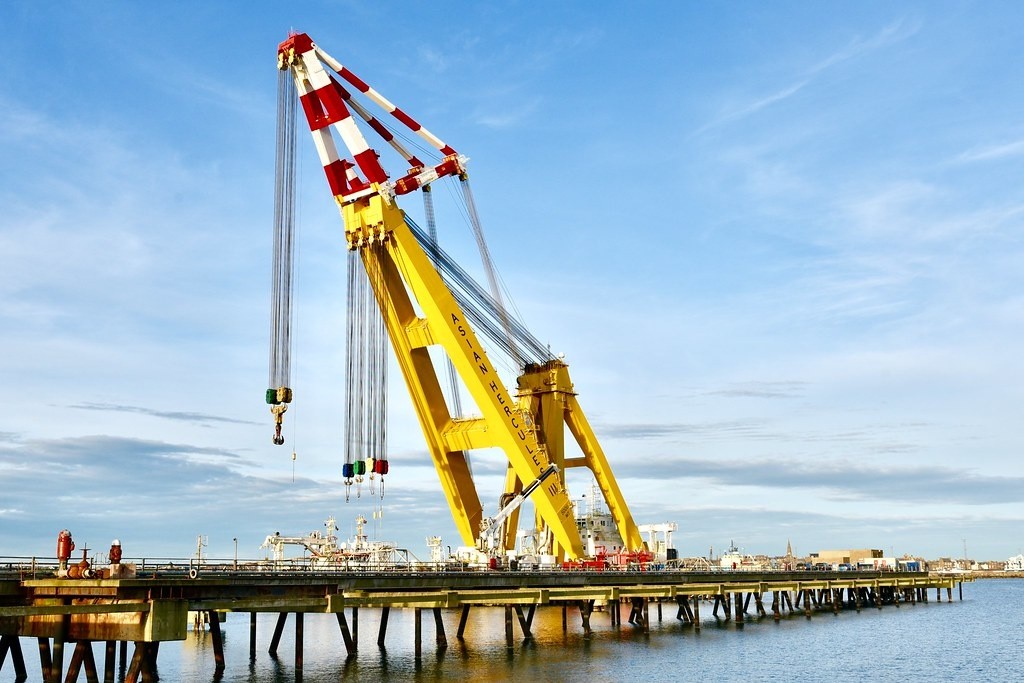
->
[838,563,856,571]
[815,562,832,570]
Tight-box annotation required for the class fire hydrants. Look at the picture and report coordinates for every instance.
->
[109,538,122,564]
[57,529,75,578]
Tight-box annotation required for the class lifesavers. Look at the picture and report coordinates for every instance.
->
[189,569,198,579]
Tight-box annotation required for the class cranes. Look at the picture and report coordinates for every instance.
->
[265,25,657,575]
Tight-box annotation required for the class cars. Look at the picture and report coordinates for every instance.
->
[796,563,811,570]
